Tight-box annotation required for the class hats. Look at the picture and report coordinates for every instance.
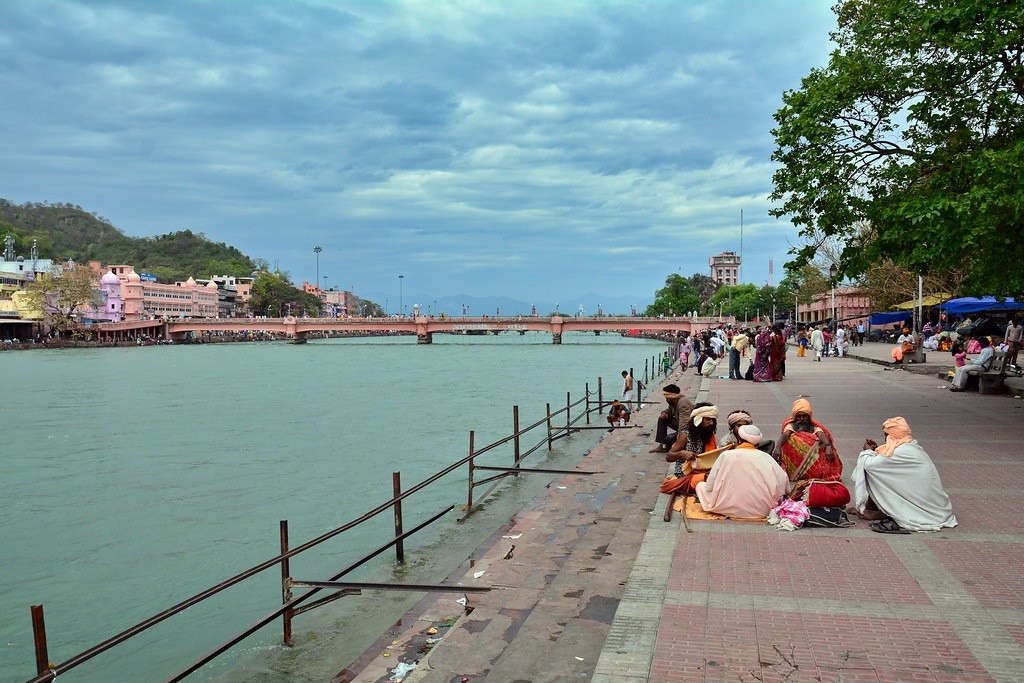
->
[792,399,813,420]
[875,417,912,457]
[738,425,763,445]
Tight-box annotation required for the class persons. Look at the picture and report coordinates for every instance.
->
[662,314,1024,394]
[622,370,634,411]
[719,409,774,456]
[660,401,718,495]
[607,399,631,433]
[772,397,843,482]
[694,424,790,519]
[648,384,695,453]
[850,416,959,534]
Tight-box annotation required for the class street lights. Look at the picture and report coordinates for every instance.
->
[830,261,838,325]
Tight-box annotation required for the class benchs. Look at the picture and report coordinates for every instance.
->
[969,351,1009,394]
[903,334,923,365]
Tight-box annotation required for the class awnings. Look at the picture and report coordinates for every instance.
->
[866,289,1024,325]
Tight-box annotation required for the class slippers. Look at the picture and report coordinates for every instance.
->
[869,518,912,534]
